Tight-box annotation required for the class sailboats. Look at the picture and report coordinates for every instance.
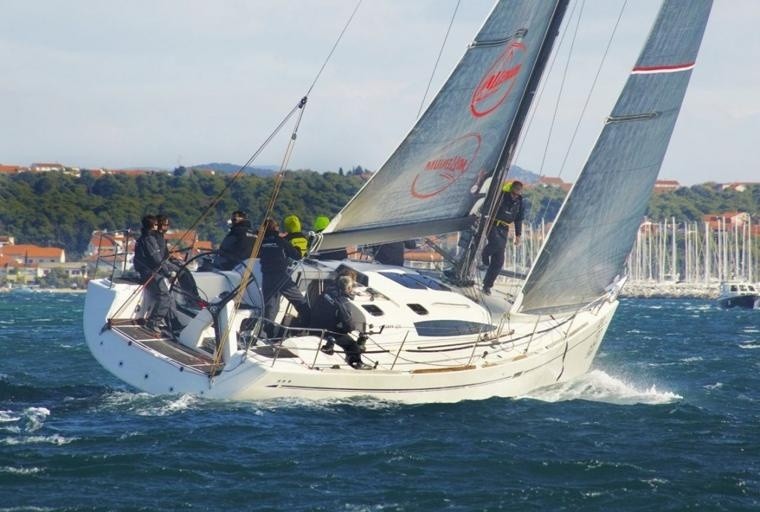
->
[81,0,714,403]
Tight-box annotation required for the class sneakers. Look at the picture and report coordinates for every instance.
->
[482,285,492,294]
[322,346,333,355]
[350,362,372,370]
[144,323,162,334]
[483,256,490,267]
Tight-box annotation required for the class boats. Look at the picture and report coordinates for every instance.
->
[712,283,760,309]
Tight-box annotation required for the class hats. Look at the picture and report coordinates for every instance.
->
[313,217,329,232]
[284,215,301,233]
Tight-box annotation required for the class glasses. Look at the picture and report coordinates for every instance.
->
[232,218,243,221]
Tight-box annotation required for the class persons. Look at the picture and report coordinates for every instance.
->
[134,215,186,334]
[482,180,525,294]
[155,215,210,309]
[214,212,371,369]
[373,239,416,267]
